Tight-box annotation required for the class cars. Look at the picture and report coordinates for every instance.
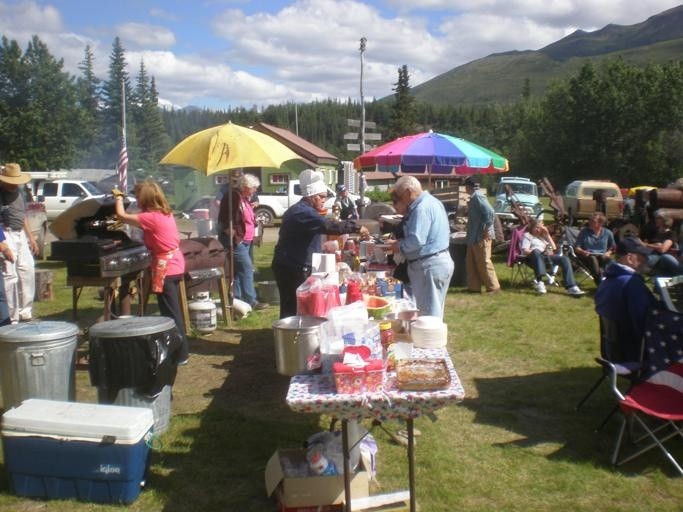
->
[494,177,544,222]
[23,182,46,260]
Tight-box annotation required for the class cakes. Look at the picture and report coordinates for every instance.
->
[395,358,451,390]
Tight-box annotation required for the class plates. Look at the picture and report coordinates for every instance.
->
[411,316,447,349]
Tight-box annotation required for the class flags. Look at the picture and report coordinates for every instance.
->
[115,85,128,191]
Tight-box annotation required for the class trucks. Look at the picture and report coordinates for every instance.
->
[20,171,135,230]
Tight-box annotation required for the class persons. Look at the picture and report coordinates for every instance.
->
[463,177,501,296]
[218,173,270,310]
[335,183,359,222]
[0,216,15,326]
[518,209,682,366]
[111,182,190,367]
[270,192,371,318]
[383,175,455,321]
[215,169,259,263]
[0,163,40,326]
[386,190,412,296]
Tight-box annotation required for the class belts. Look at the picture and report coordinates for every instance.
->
[407,248,450,264]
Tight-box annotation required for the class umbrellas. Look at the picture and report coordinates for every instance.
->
[352,130,508,192]
[159,121,299,319]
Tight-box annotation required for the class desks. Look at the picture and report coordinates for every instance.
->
[66,270,146,366]
[286,260,466,512]
[178,267,231,337]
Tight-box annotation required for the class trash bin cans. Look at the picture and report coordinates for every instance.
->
[88,315,189,434]
[0,321,80,413]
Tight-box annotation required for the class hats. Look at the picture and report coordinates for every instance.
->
[616,236,654,257]
[337,184,347,192]
[462,176,479,186]
[299,168,327,198]
[0,163,32,185]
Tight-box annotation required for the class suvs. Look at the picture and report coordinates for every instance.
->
[554,179,624,226]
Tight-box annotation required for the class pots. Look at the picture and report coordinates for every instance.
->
[254,219,263,237]
[197,218,213,236]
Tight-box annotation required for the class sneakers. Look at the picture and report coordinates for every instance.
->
[253,301,269,311]
[484,287,502,294]
[176,359,188,366]
[565,285,588,296]
[462,289,481,294]
[533,279,548,295]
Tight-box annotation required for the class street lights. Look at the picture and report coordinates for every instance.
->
[357,34,367,218]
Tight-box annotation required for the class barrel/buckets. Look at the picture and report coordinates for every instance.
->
[255,281,280,306]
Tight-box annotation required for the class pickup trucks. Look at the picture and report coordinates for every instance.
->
[253,179,375,227]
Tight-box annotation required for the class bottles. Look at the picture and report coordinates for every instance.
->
[379,320,395,352]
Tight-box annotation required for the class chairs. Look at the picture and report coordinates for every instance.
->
[594,357,683,476]
[574,314,647,433]
[566,226,606,285]
[509,227,557,289]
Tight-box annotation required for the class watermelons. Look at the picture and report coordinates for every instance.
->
[366,298,388,319]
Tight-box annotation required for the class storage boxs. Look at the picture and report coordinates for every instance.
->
[1,398,154,505]
[264,447,374,512]
